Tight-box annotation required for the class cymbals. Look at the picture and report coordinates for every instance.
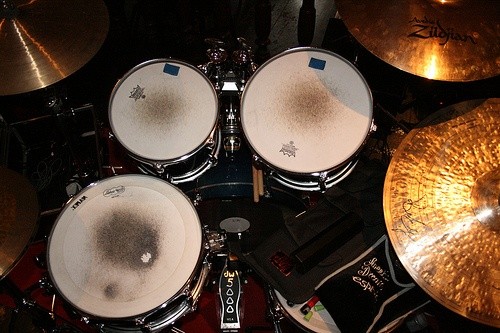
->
[382,98,500,328]
[333,0,500,83]
[0,1,111,96]
[0,168,39,280]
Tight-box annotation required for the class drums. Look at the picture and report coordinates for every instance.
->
[110,60,222,182]
[46,174,202,318]
[240,47,372,191]
